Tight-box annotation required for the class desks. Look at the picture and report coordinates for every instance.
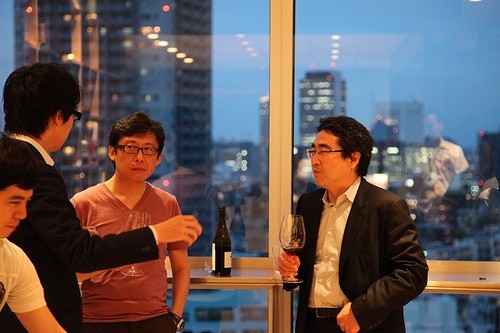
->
[166,256,500,333]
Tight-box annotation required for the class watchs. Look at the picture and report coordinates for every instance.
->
[169,312,185,331]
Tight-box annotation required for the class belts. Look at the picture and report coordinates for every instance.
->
[307,307,342,318]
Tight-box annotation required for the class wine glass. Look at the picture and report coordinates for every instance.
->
[278,214,306,283]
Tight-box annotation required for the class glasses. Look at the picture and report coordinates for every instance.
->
[72,111,82,120]
[306,148,346,159]
[115,145,160,155]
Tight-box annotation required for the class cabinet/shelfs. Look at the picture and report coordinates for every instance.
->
[24,0,133,197]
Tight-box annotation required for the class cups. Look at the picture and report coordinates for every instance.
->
[82,226,96,237]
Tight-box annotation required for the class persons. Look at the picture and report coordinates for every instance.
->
[277,116,429,333]
[0,62,202,333]
[417,113,500,224]
[150,145,220,257]
[0,131,67,333]
[69,112,190,333]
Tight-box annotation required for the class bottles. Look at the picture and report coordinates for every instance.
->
[212,206,232,277]
